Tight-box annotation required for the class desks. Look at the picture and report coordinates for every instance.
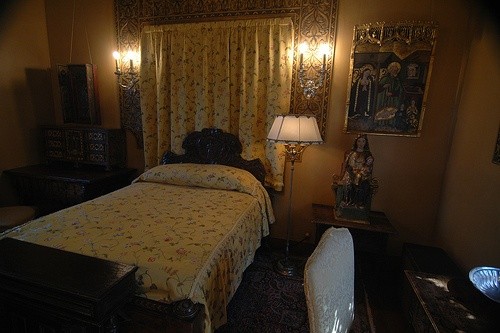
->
[3,164,131,217]
[403,269,500,333]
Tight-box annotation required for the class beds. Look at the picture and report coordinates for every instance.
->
[0,128,277,333]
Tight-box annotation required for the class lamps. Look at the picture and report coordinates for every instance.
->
[267,115,324,276]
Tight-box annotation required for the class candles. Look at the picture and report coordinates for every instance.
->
[127,51,135,69]
[113,51,120,71]
[320,45,328,68]
[299,44,307,67]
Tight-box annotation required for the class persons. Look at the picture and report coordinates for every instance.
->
[330,134,379,225]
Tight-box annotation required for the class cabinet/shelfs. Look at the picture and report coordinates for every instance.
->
[58,64,96,127]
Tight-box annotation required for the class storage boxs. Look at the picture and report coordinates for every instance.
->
[38,124,125,172]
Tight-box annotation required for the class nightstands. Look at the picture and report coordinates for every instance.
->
[312,203,395,246]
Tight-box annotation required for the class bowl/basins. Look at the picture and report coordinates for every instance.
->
[468,266,500,304]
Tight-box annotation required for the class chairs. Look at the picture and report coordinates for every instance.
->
[303,226,354,333]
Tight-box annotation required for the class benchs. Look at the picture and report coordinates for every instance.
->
[0,237,138,333]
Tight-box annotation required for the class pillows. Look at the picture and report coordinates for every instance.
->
[131,164,259,199]
